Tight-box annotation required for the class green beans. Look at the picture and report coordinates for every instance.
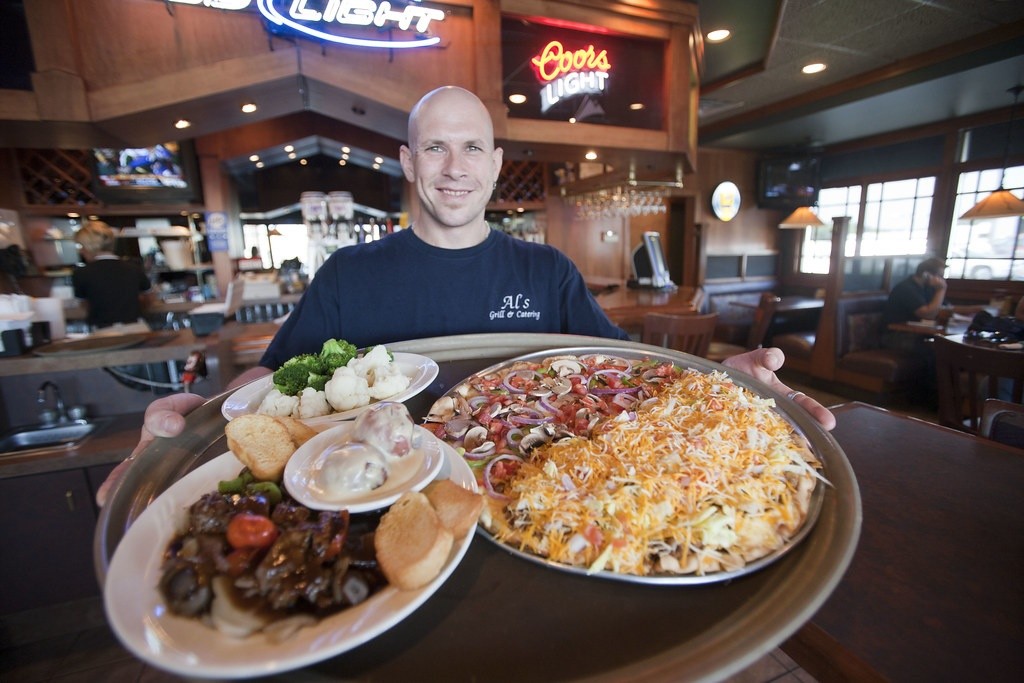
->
[218,473,284,505]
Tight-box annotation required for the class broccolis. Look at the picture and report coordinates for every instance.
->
[257,339,411,418]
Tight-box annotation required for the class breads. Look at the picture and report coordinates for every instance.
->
[224,413,326,480]
[375,478,484,591]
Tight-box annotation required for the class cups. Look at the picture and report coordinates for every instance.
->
[31,321,51,349]
[1,329,27,357]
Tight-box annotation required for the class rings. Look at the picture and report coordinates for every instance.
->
[788,391,805,398]
[125,455,135,460]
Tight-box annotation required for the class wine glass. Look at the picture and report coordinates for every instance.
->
[570,187,668,222]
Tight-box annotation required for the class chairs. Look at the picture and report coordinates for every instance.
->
[642,311,720,359]
[934,334,1024,435]
[708,291,781,363]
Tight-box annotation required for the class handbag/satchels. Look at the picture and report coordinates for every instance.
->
[964,309,1024,345]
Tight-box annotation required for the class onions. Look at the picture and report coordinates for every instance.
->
[453,355,641,498]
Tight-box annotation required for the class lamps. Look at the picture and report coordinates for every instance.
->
[779,207,824,228]
[959,85,1024,219]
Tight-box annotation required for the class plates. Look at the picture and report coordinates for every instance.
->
[284,423,442,514]
[221,351,439,428]
[425,346,823,586]
[101,435,481,680]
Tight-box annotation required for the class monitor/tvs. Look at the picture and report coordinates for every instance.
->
[89,138,202,206]
[756,155,820,207]
[644,232,671,286]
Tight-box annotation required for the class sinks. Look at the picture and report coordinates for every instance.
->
[0,422,96,459]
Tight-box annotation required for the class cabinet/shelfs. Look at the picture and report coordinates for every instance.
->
[0,461,124,615]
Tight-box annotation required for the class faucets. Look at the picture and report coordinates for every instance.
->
[37,381,68,424]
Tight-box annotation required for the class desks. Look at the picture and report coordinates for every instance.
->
[779,400,1024,682]
[729,296,825,312]
[885,319,954,335]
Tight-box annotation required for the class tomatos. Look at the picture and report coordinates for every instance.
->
[226,514,277,549]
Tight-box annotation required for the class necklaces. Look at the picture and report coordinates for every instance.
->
[411,220,490,237]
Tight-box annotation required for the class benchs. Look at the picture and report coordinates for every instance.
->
[709,292,924,394]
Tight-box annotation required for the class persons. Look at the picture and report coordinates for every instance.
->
[97,86,836,508]
[0,223,28,294]
[880,259,951,407]
[74,221,153,328]
[96,145,183,185]
